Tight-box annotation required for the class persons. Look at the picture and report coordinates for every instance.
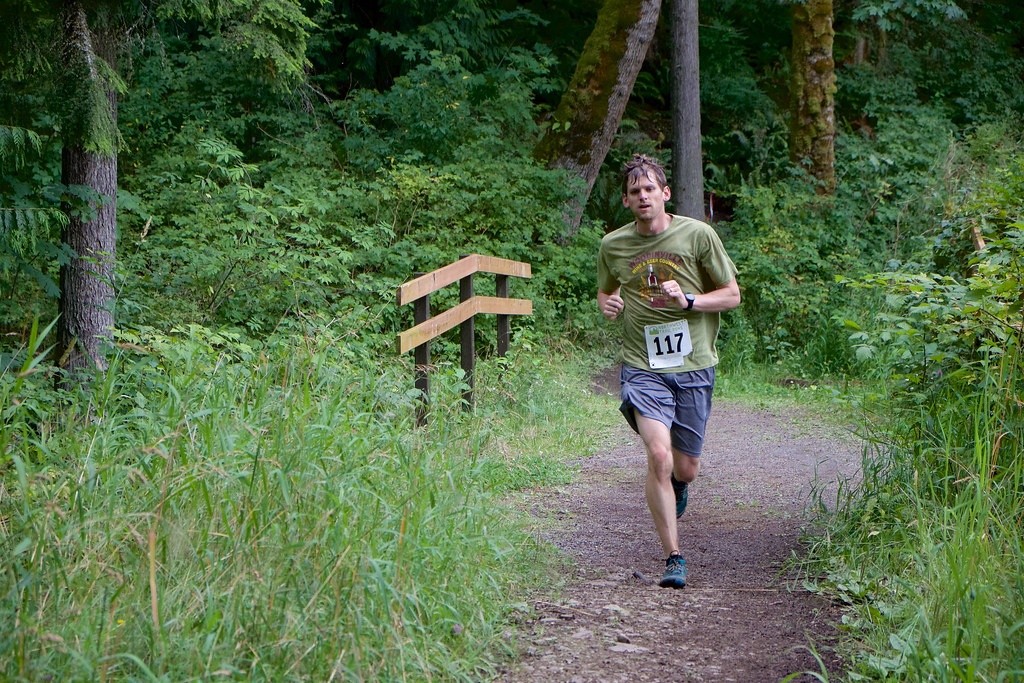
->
[596,154,742,590]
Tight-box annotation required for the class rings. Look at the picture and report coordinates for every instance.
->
[670,288,673,292]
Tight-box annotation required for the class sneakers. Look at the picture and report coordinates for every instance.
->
[660,549,686,587]
[674,484,688,518]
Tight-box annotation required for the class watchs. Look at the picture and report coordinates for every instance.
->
[682,294,695,311]
